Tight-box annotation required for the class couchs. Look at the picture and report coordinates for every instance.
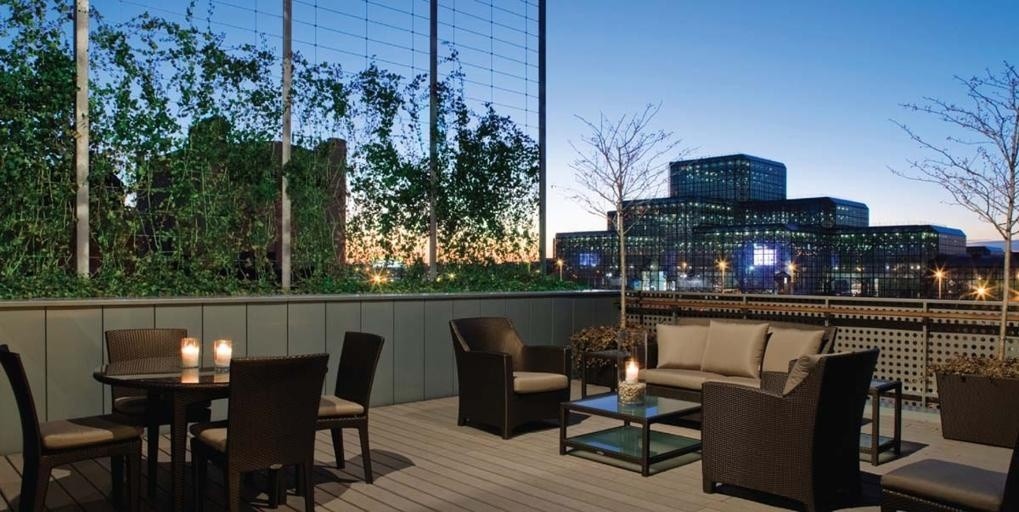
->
[631,316,837,423]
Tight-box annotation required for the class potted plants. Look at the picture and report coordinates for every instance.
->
[551,100,701,387]
[886,59,1019,449]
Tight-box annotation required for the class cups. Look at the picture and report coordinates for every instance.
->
[625,361,639,383]
[214,338,232,372]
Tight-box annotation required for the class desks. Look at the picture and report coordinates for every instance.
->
[93,357,231,511]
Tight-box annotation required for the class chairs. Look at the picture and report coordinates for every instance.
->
[0,344,145,512]
[449,317,572,439]
[701,348,880,512]
[106,329,211,510]
[190,354,330,512]
[295,331,385,496]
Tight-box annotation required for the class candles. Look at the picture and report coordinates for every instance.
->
[213,339,232,368]
[625,357,639,385]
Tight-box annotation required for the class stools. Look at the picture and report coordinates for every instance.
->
[880,438,1015,511]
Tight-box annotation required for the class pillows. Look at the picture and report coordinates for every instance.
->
[700,319,769,379]
[655,323,709,368]
[782,351,853,396]
[761,327,825,372]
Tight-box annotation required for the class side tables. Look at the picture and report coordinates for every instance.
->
[578,349,630,400]
[858,378,902,466]
